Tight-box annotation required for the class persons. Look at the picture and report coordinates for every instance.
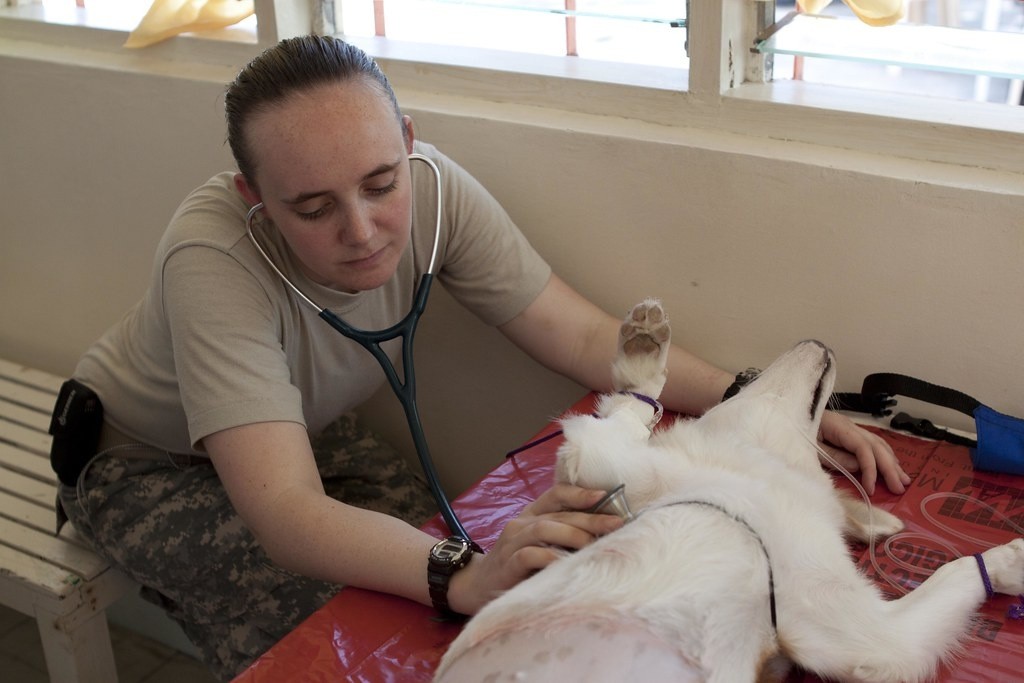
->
[49,34,911,683]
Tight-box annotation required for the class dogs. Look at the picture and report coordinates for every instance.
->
[433,300,1024,683]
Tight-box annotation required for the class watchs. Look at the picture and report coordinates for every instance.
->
[427,536,485,613]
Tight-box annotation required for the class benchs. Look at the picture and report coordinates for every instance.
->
[1,358,126,683]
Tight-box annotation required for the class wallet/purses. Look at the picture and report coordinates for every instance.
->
[49,379,104,488]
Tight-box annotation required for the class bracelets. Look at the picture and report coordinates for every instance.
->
[722,366,763,402]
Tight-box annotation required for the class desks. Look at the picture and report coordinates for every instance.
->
[226,367,1023,681]
